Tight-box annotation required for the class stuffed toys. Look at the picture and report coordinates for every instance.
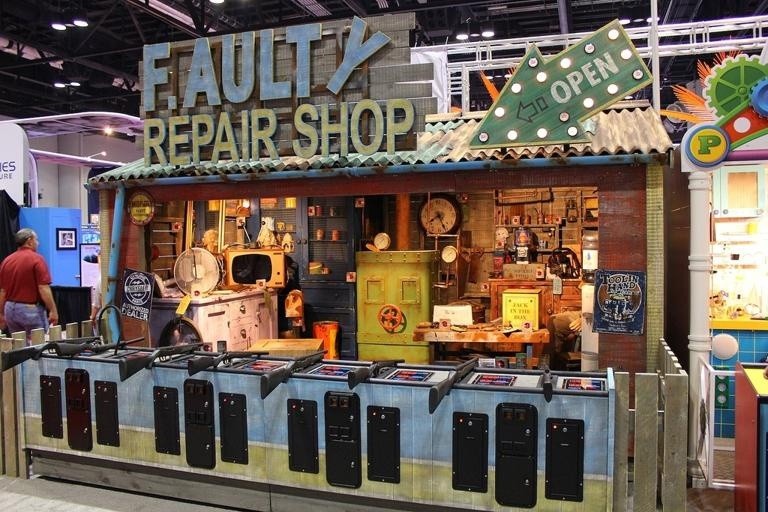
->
[495,227,509,251]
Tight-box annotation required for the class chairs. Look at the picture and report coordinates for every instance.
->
[549,310,582,371]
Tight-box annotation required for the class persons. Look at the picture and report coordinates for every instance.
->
[0,228,59,346]
[89,254,102,337]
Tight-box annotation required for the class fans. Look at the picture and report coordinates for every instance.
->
[172,247,221,296]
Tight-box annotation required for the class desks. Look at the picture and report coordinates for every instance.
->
[421,325,550,367]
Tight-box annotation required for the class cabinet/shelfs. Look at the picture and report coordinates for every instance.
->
[185,196,255,250]
[496,220,563,255]
[149,292,281,350]
[707,160,765,220]
[256,196,355,281]
[490,282,584,336]
[301,285,354,358]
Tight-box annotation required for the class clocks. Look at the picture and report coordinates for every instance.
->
[416,195,464,237]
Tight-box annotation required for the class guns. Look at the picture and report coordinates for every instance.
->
[259,350,328,399]
[542,364,553,402]
[119,342,212,381]
[348,360,405,390]
[187,350,269,376]
[0,337,100,372]
[53,337,145,356]
[429,357,478,413]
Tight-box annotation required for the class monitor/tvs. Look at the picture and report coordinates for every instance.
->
[225,249,285,288]
